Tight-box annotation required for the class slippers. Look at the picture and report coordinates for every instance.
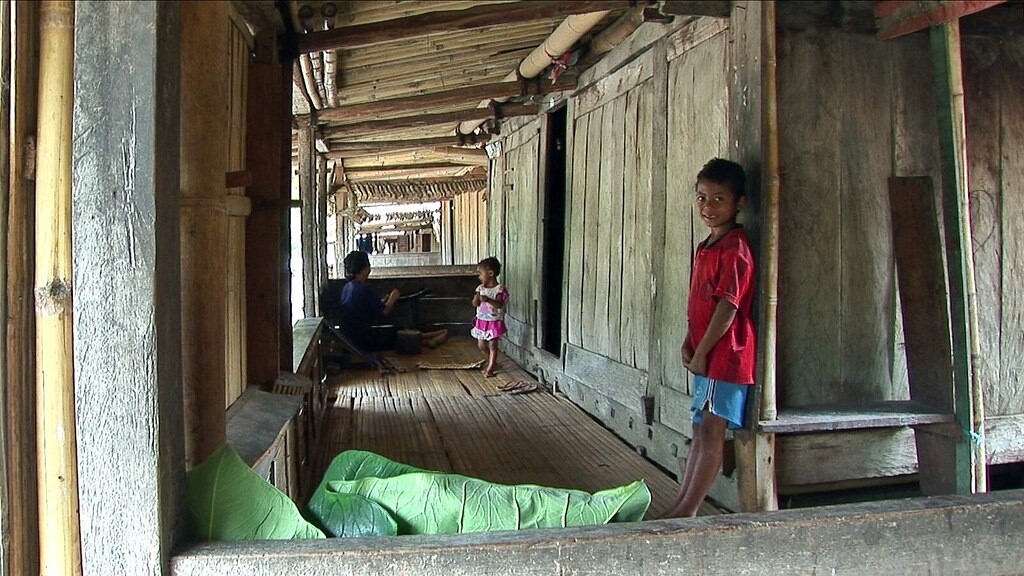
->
[508,383,539,395]
[496,380,526,391]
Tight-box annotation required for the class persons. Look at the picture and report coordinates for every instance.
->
[338,251,449,354]
[470,257,509,378]
[652,158,757,520]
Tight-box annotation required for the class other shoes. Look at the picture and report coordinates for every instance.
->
[326,360,339,375]
